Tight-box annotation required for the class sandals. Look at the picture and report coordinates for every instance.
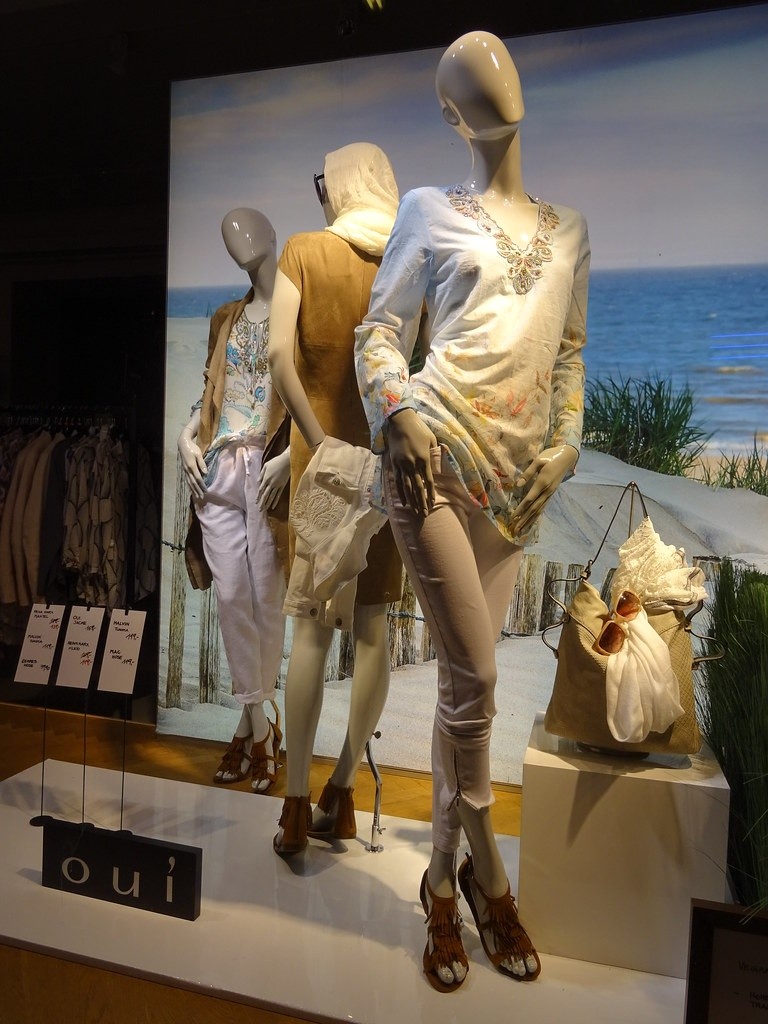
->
[273,790,313,852]
[419,868,469,995]
[458,852,543,982]
[250,717,283,795]
[306,777,356,840]
[213,735,252,784]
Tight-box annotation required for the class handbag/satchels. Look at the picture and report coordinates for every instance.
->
[539,481,728,756]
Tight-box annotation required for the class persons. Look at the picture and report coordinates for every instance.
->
[177,208,290,794]
[269,142,397,858]
[353,31,591,995]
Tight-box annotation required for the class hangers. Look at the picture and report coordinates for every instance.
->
[0,402,128,445]
